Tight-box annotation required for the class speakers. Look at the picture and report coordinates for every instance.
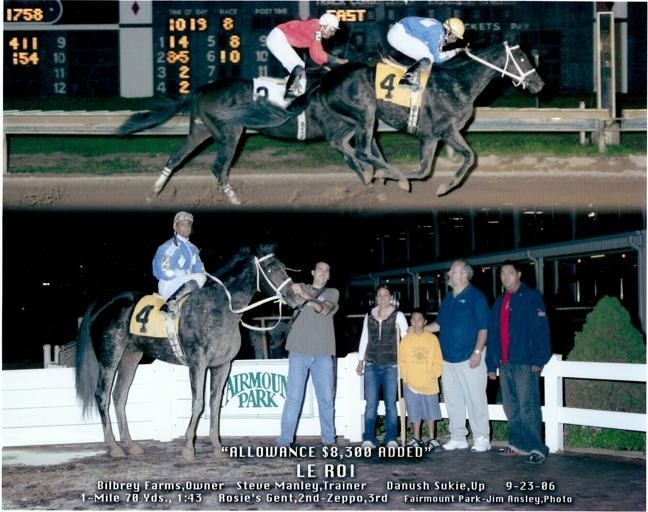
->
[284,64,303,101]
[397,50,431,90]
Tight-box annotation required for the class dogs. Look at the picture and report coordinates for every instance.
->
[72,243,307,461]
[116,38,402,209]
[210,28,550,198]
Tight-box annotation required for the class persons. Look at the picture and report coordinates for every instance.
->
[407,259,491,453]
[386,16,465,89]
[485,260,552,465]
[276,261,340,449]
[150,210,207,318]
[397,310,443,449]
[266,13,350,101]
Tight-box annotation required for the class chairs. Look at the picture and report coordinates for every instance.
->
[360,440,376,452]
[443,439,469,451]
[524,448,547,464]
[386,441,400,450]
[470,440,490,452]
[404,437,423,451]
[492,443,524,455]
[159,304,177,320]
[425,438,442,453]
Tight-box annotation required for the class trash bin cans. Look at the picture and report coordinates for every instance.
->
[473,349,482,354]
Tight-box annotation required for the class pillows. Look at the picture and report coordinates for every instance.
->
[443,16,464,42]
[318,12,342,31]
[173,210,194,229]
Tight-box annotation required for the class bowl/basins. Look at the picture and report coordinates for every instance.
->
[356,284,409,451]
